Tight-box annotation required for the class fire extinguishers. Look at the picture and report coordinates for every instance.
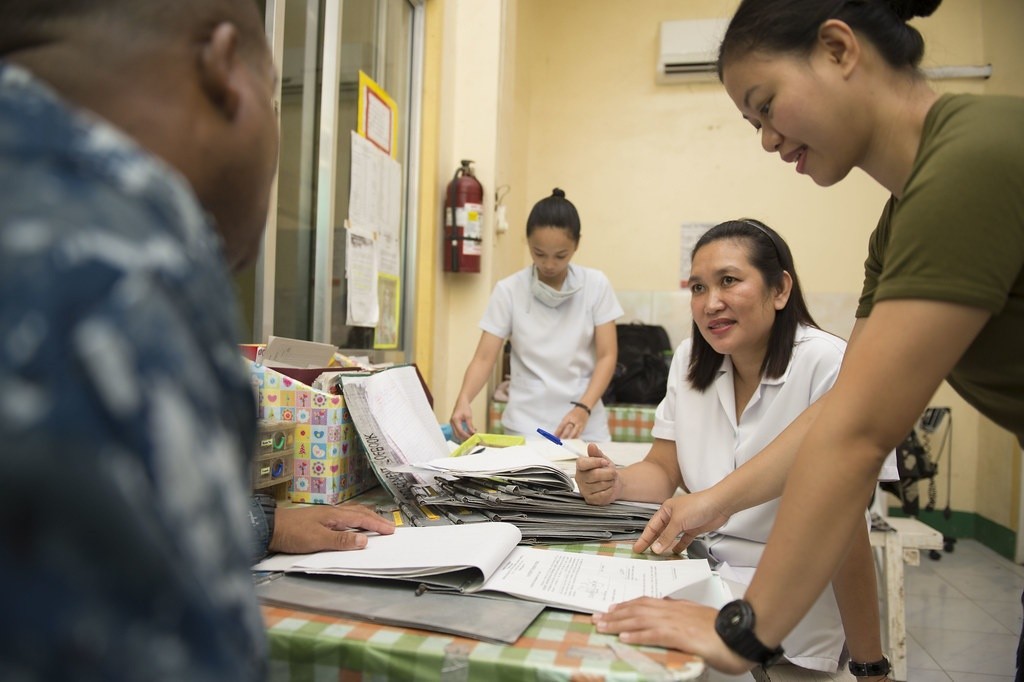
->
[442,158,485,273]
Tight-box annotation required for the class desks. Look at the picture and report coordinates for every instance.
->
[248,479,709,682]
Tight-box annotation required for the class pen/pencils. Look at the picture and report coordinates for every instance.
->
[416,584,426,597]
[537,428,588,459]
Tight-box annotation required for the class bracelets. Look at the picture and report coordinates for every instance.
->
[570,401,591,415]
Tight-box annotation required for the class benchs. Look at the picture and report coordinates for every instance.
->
[866,517,943,682]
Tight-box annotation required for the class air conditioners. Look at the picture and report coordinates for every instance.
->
[656,17,729,84]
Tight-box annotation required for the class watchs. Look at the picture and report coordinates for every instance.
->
[715,599,784,664]
[848,652,892,677]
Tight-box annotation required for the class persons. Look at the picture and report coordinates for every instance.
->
[0,0,396,682]
[589,0,1024,682]
[449,186,626,443]
[574,216,900,682]
